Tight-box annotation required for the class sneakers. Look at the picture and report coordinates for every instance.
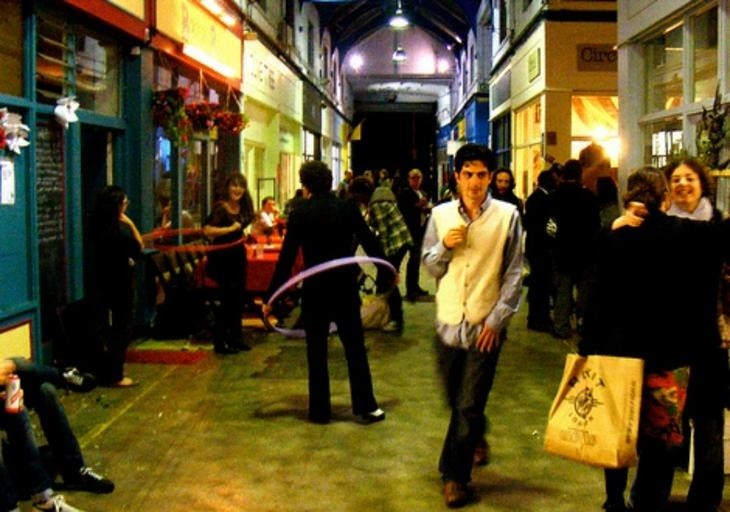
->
[215,342,251,354]
[65,467,114,493]
[383,321,397,332]
[32,493,81,512]
[58,368,96,393]
[369,408,384,417]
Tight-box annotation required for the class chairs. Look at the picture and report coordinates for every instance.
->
[152,240,219,323]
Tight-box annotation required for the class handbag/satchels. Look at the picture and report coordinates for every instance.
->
[544,354,645,469]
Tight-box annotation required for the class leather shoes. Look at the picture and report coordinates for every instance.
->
[115,377,139,385]
[474,443,488,467]
[443,481,465,506]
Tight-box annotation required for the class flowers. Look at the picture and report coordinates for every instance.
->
[0,108,30,153]
[150,86,246,148]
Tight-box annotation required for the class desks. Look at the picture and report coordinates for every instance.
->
[195,242,304,329]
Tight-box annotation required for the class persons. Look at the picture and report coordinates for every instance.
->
[154,193,178,229]
[1,355,115,495]
[284,166,432,333]
[253,197,280,235]
[490,143,619,338]
[417,142,524,508]
[575,165,729,511]
[259,161,401,427]
[1,386,86,511]
[611,155,730,511]
[203,172,254,356]
[83,185,147,389]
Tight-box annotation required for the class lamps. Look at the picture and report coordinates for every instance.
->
[388,0,410,28]
[392,44,407,61]
[179,42,233,78]
